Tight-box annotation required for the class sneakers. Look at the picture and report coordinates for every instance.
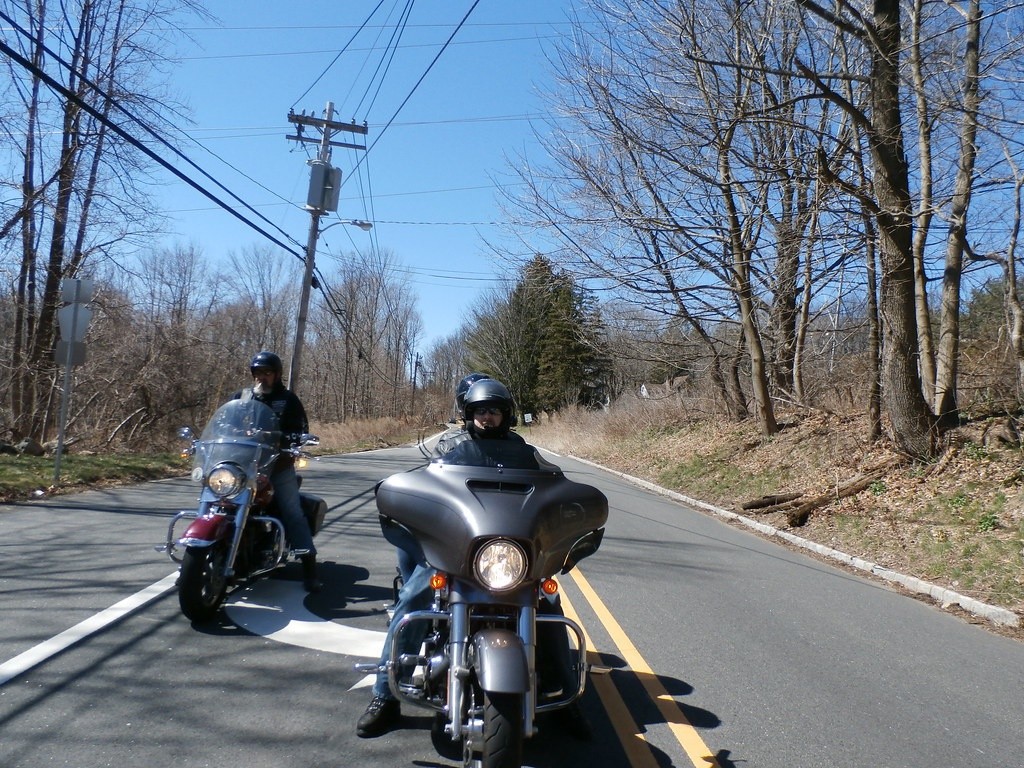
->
[356,697,401,737]
[550,700,592,740]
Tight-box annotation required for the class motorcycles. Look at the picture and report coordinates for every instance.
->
[155,398,323,623]
[373,460,610,768]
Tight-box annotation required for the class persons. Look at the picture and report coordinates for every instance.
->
[356,373,569,737]
[213,352,324,593]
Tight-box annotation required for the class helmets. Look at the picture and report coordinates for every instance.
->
[461,379,517,431]
[249,352,283,384]
[455,374,491,417]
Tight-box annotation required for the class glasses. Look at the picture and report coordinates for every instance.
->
[253,368,273,375]
[474,406,501,415]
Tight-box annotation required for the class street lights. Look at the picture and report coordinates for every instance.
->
[284,219,374,397]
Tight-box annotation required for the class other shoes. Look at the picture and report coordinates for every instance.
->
[302,558,323,592]
